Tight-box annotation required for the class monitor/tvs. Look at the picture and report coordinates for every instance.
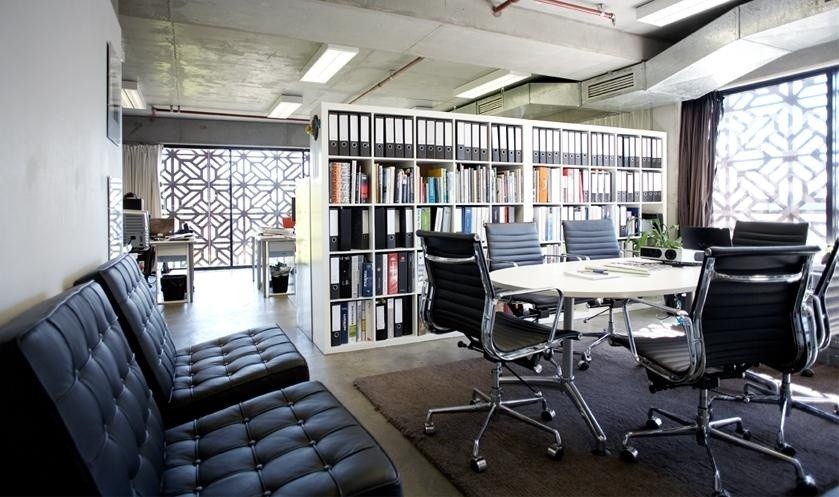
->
[149,218,175,240]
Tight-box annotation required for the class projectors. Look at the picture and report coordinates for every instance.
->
[639,246,706,267]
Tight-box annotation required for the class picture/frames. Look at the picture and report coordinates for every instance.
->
[105,39,124,147]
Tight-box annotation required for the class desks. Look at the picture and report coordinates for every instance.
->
[486,257,780,458]
[148,231,197,306]
[252,233,297,299]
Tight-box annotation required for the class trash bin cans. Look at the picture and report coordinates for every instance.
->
[270,271,290,293]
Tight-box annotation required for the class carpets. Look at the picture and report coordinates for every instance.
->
[351,336,839,497]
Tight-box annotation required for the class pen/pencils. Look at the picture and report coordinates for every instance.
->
[576,269,603,274]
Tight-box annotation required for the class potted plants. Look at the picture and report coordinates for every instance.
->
[623,217,683,317]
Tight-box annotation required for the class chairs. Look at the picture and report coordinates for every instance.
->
[72,252,310,428]
[412,227,564,472]
[708,239,839,459]
[622,244,817,497]
[561,218,640,365]
[0,279,402,497]
[482,220,590,375]
[730,219,809,393]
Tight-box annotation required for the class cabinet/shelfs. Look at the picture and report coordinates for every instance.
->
[311,101,529,357]
[530,119,668,326]
[294,177,312,342]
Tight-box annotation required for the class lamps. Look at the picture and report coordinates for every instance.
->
[121,80,147,111]
[452,69,532,100]
[265,94,303,121]
[298,44,360,85]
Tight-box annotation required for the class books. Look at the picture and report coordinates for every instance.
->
[341,296,412,343]
[533,166,588,203]
[417,207,515,240]
[340,253,412,298]
[535,206,640,264]
[330,160,524,204]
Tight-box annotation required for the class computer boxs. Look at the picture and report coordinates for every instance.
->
[123,210,150,251]
